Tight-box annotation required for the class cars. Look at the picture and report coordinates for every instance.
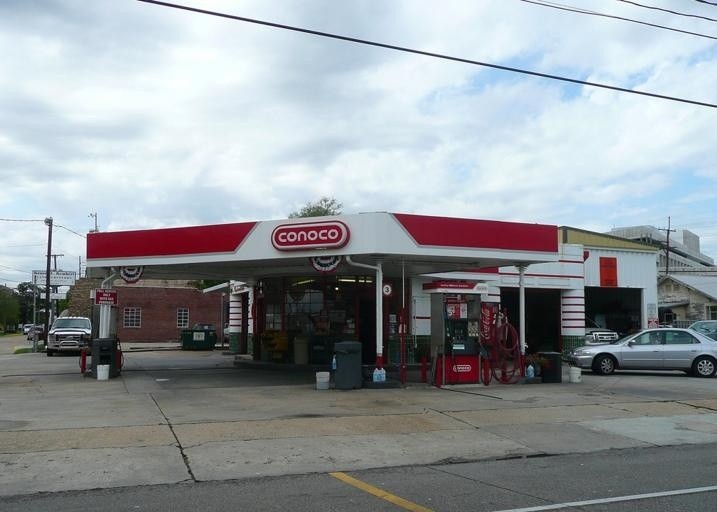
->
[567,326,717,379]
[658,324,684,342]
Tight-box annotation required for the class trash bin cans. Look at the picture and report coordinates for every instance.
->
[334,341,362,389]
[539,352,563,383]
[90,338,117,378]
[292,337,310,365]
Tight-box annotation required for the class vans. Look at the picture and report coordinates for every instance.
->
[22,323,34,334]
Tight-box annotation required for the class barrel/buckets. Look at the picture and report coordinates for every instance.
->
[570,366,582,382]
[315,371,330,390]
[373,368,386,382]
[526,365,535,377]
[96,364,110,381]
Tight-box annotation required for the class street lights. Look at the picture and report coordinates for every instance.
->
[40,217,57,345]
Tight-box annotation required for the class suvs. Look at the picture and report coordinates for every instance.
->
[46,315,94,358]
[26,326,44,340]
[688,320,717,342]
[583,314,620,345]
